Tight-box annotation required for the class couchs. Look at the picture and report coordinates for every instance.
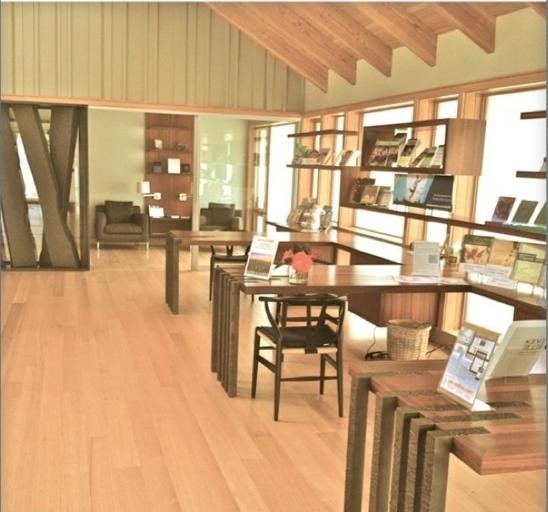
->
[200,204,240,232]
[93,201,150,258]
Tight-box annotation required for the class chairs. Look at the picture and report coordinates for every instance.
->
[250,297,348,421]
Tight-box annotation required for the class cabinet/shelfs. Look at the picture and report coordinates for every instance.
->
[361,119,486,176]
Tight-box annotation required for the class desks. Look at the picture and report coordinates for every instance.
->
[343,360,548,511]
[211,261,469,400]
[166,230,338,313]
